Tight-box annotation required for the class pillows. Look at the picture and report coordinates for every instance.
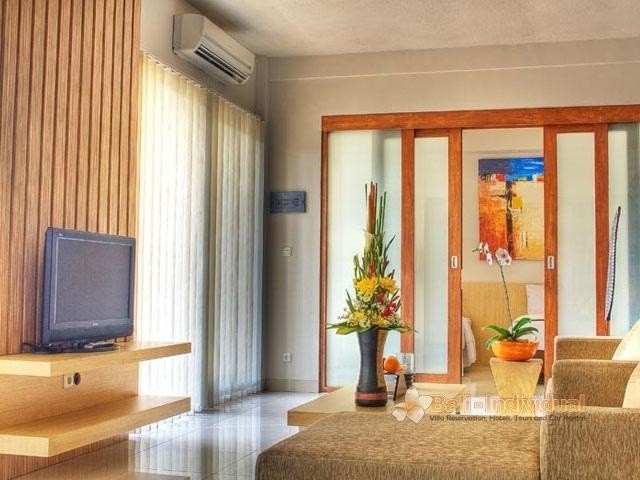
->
[525,284,545,315]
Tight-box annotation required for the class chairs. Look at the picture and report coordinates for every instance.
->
[541,317,640,480]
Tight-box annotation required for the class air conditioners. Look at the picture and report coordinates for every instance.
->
[172,13,256,88]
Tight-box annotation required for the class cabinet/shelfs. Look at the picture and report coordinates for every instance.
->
[0,341,193,459]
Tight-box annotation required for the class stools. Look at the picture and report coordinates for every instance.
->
[254,408,543,479]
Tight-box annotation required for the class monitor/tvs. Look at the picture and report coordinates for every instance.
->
[41,228,136,353]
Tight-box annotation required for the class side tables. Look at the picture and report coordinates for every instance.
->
[490,357,544,401]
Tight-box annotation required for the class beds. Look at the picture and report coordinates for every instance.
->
[508,314,544,383]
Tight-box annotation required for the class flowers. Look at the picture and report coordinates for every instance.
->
[470,241,540,348]
[322,177,421,337]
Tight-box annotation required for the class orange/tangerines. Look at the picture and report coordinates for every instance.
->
[384,355,400,373]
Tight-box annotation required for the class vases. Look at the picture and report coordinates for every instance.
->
[355,329,389,407]
[491,341,539,361]
